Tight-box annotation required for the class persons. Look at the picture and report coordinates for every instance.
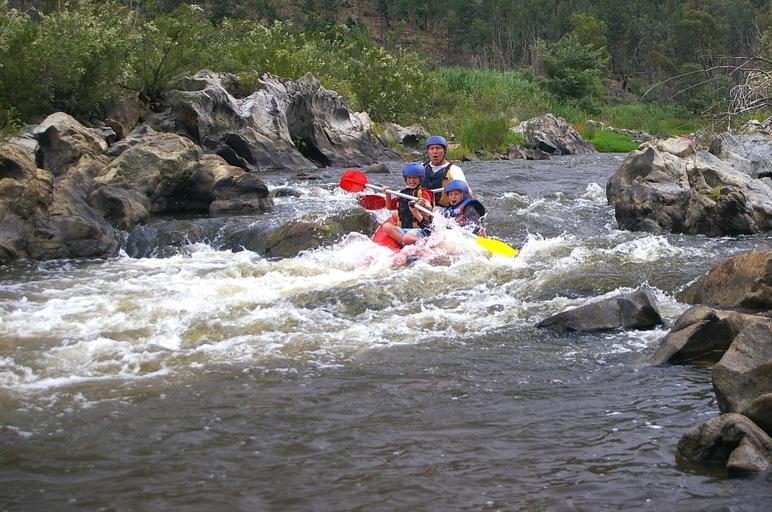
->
[382,161,435,244]
[402,179,487,245]
[420,135,473,207]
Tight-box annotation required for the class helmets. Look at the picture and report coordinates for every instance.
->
[444,179,469,196]
[403,161,427,186]
[426,134,449,148]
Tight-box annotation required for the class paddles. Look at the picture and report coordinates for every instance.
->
[340,170,420,202]
[410,201,517,258]
[358,187,444,211]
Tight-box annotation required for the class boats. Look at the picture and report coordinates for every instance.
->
[365,213,486,272]
